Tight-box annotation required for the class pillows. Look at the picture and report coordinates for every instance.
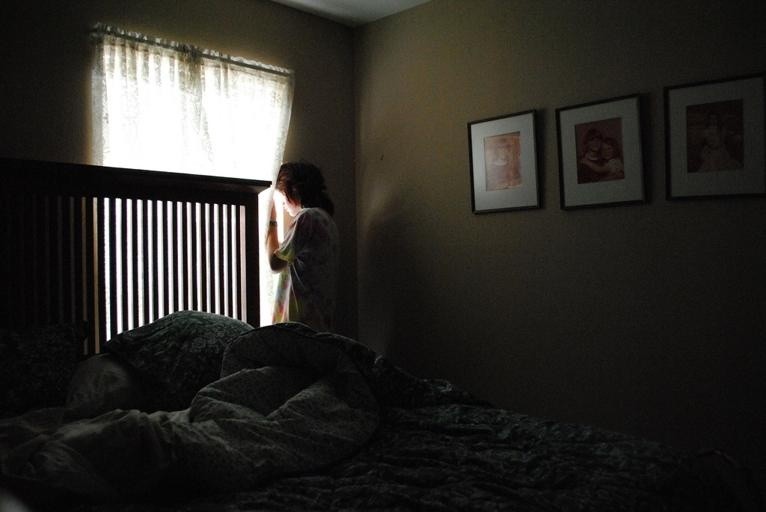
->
[0,307,266,422]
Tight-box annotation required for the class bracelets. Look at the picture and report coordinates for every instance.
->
[269,221,278,226]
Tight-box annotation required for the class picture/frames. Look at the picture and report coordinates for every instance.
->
[554,91,648,211]
[664,72,766,200]
[467,108,542,215]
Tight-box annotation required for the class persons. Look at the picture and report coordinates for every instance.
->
[488,139,511,190]
[691,113,739,172]
[265,161,341,330]
[579,130,623,181]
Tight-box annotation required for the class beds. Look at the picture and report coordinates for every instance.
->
[0,158,766,512]
